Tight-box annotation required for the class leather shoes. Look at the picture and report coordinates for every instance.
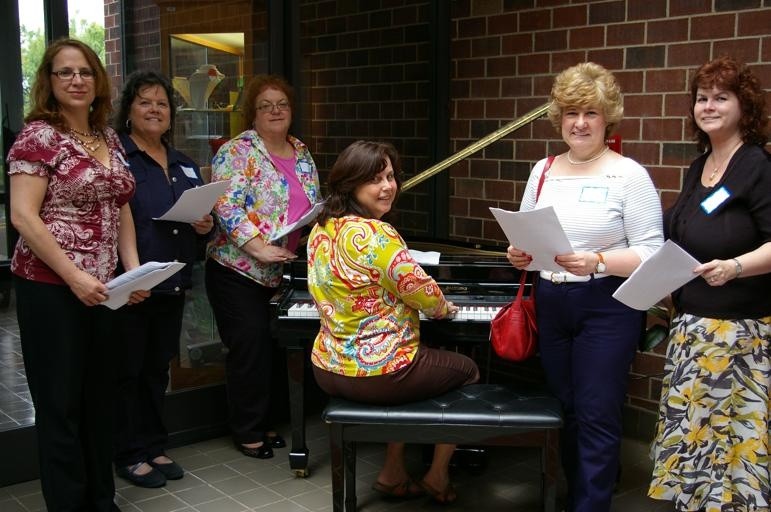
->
[233,440,273,459]
[267,436,286,448]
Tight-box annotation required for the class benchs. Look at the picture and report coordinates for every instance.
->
[321,384,563,512]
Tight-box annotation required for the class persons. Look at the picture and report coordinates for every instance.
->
[306,139,480,504]
[113,68,217,489]
[506,61,665,511]
[648,54,771,512]
[6,38,152,511]
[205,74,322,461]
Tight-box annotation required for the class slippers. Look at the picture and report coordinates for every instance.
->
[417,479,457,503]
[372,478,424,497]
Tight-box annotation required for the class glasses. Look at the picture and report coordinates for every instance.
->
[256,101,290,112]
[52,70,97,80]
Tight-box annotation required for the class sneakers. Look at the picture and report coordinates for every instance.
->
[148,454,184,480]
[115,461,167,488]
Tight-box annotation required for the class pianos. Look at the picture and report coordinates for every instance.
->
[269,242,541,477]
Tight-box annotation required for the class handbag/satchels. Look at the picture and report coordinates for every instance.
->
[488,299,539,361]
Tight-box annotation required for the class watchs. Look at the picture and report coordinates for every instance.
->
[732,258,743,278]
[594,250,606,275]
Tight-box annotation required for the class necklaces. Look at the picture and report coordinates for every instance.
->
[709,139,742,180]
[566,144,609,165]
[69,127,102,152]
